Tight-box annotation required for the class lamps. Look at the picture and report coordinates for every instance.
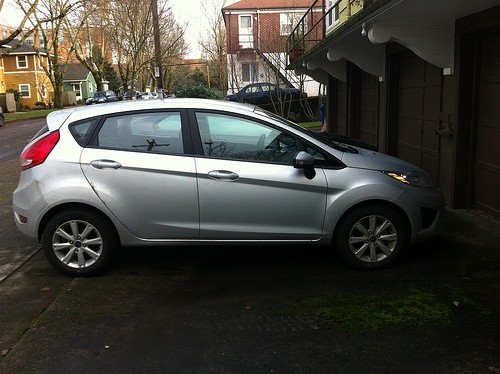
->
[361,23,367,36]
[326,51,342,62]
[302,60,306,67]
[306,62,318,70]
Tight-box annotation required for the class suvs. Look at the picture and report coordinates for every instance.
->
[13,97,445,275]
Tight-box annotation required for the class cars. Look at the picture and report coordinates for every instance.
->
[85,89,175,105]
[224,83,307,105]
